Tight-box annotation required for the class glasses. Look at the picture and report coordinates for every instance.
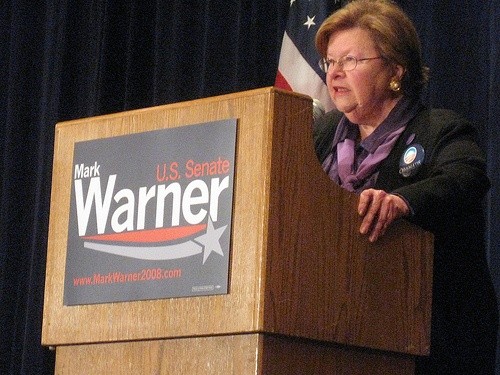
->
[318,55,385,73]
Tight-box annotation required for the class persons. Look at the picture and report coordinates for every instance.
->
[312,0,497,375]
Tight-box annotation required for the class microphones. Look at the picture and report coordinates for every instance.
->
[314,99,324,123]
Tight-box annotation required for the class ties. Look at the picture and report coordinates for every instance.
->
[336,124,409,192]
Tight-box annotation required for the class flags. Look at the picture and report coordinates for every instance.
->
[274,0,337,121]
